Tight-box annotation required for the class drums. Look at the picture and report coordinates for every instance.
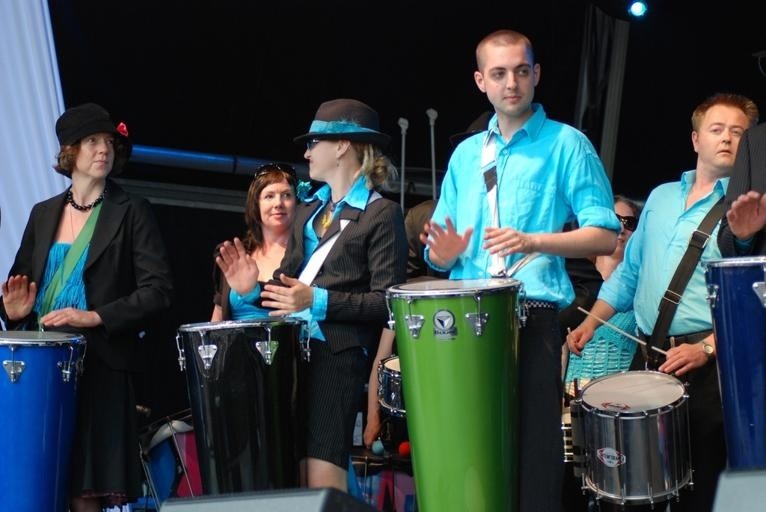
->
[0,330,87,512]
[701,256,766,472]
[561,423,574,463]
[377,356,407,418]
[385,277,528,512]
[569,369,695,505]
[176,318,313,495]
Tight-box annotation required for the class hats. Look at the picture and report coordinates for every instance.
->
[293,99,394,151]
[56,102,132,163]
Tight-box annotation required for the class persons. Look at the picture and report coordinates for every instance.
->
[594,195,643,281]
[419,31,622,510]
[204,164,328,321]
[565,93,760,512]
[214,100,411,496]
[716,122,766,260]
[362,109,569,454]
[0,103,178,511]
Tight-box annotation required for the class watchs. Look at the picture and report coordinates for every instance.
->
[700,340,716,364]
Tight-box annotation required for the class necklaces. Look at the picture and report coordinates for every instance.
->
[67,186,109,212]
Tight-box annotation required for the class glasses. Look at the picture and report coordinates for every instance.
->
[616,213,639,231]
[255,162,296,178]
[306,136,322,149]
